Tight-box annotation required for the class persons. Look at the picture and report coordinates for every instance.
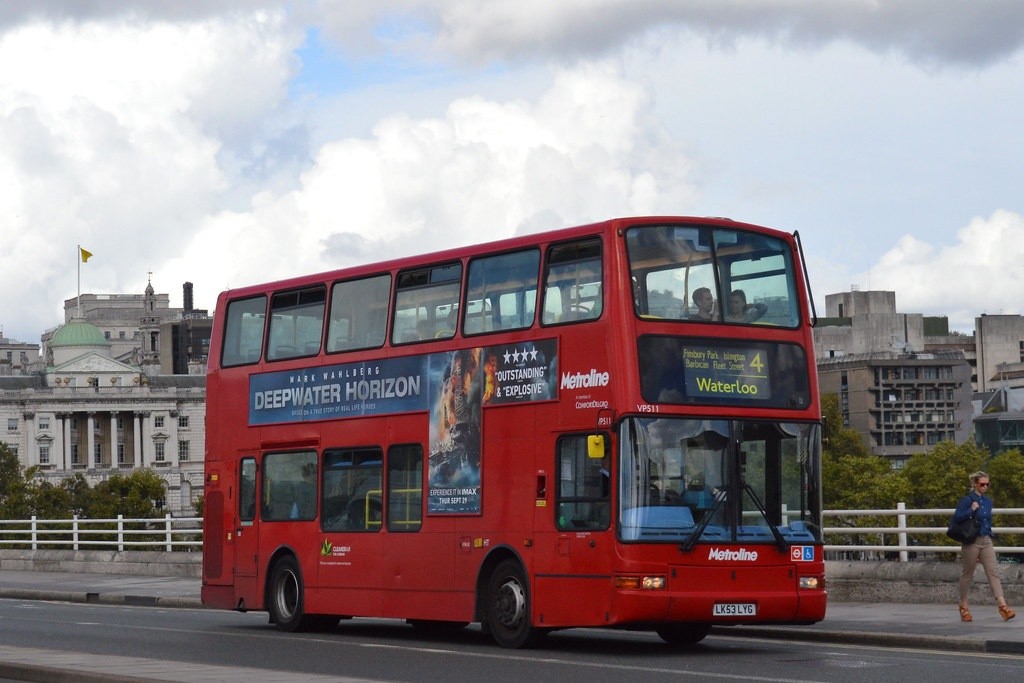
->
[950,471,1016,622]
[447,308,468,337]
[687,288,719,321]
[724,290,768,323]
[415,319,433,339]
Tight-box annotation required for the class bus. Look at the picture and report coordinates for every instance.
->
[200,213,830,649]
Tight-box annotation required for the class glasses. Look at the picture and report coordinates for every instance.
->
[980,482,990,487]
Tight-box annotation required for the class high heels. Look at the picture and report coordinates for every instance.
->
[999,605,1015,621]
[960,606,972,621]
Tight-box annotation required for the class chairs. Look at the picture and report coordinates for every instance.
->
[561,305,590,322]
[246,329,454,365]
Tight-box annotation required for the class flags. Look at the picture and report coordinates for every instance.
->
[81,248,93,262]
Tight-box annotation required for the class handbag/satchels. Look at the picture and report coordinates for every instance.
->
[946,507,981,544]
